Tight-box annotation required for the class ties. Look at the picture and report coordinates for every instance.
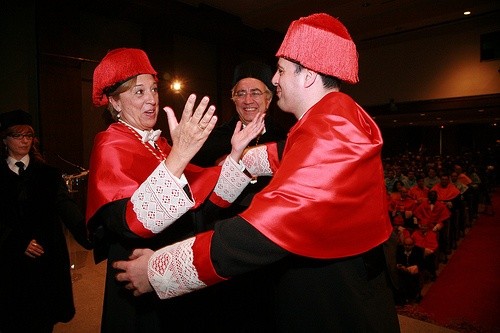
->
[16,162,24,177]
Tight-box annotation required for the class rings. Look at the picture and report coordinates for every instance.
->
[33,242,36,246]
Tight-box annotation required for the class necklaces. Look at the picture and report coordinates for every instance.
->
[247,136,259,185]
[117,119,167,163]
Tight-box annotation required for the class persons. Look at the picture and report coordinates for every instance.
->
[0,109,94,333]
[86,12,494,333]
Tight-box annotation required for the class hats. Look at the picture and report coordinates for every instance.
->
[232,60,275,93]
[276,13,359,84]
[0,108,33,129]
[91,47,157,106]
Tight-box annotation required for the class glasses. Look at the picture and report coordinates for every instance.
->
[234,91,265,100]
[7,134,33,140]
